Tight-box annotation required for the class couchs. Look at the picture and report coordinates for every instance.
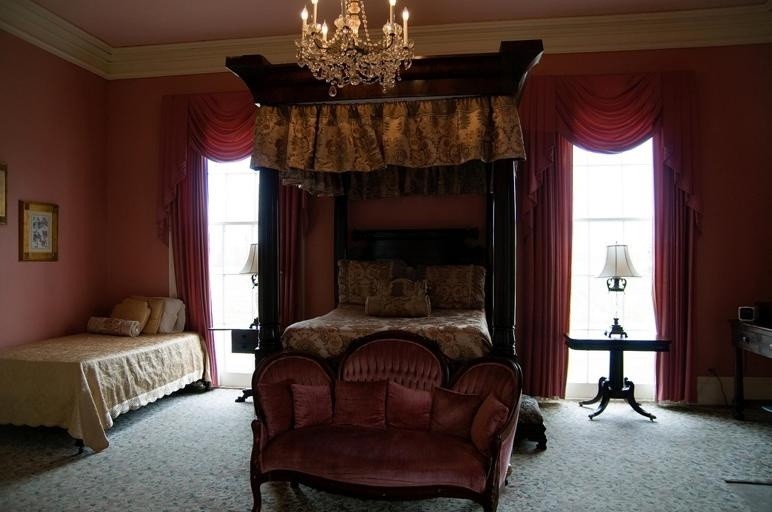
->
[250,329,524,512]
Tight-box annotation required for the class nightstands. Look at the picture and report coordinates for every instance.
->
[232,328,259,402]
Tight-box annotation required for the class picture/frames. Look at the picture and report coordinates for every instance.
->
[18,199,59,262]
[0,160,8,225]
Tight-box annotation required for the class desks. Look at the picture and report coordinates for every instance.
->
[729,319,772,421]
[565,329,672,421]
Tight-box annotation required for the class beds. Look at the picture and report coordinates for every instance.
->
[0,332,212,452]
[280,227,495,391]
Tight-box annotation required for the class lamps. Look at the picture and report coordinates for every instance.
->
[295,0,416,97]
[597,241,642,338]
[241,244,259,328]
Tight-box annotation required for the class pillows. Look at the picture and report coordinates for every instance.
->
[338,258,486,318]
[87,297,186,337]
[290,382,333,427]
[470,392,509,455]
[333,378,387,428]
[386,382,432,431]
[431,386,480,438]
[257,380,297,438]
